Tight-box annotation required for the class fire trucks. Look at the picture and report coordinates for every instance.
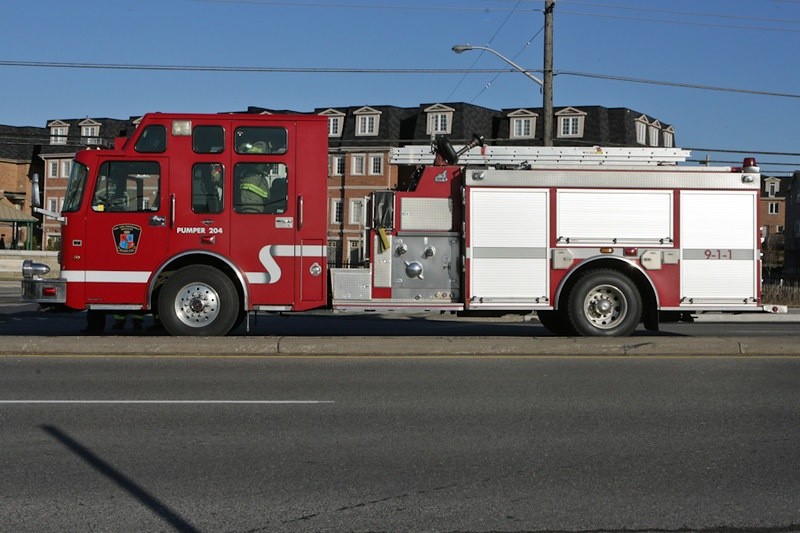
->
[21,111,788,336]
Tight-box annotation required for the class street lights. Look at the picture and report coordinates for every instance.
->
[452,44,554,147]
